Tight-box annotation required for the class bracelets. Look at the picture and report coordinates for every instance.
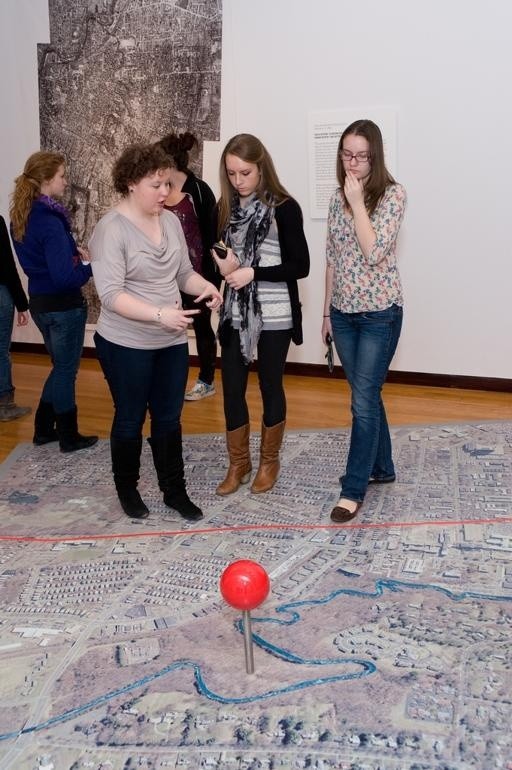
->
[155,305,164,323]
[323,314,330,318]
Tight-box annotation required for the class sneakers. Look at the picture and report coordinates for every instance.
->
[184,379,216,400]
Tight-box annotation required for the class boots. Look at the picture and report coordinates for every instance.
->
[33,408,58,445]
[251,419,285,493]
[146,433,204,521]
[53,405,98,451]
[216,423,252,496]
[111,438,150,517]
[0,386,31,421]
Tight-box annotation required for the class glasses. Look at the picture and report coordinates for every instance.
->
[337,149,370,162]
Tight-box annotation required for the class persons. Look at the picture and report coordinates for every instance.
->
[86,144,225,522]
[208,132,312,497]
[0,214,31,423]
[9,150,99,454]
[154,131,224,402]
[320,118,409,523]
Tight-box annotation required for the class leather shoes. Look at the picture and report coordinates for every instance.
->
[340,475,393,485]
[331,500,362,522]
